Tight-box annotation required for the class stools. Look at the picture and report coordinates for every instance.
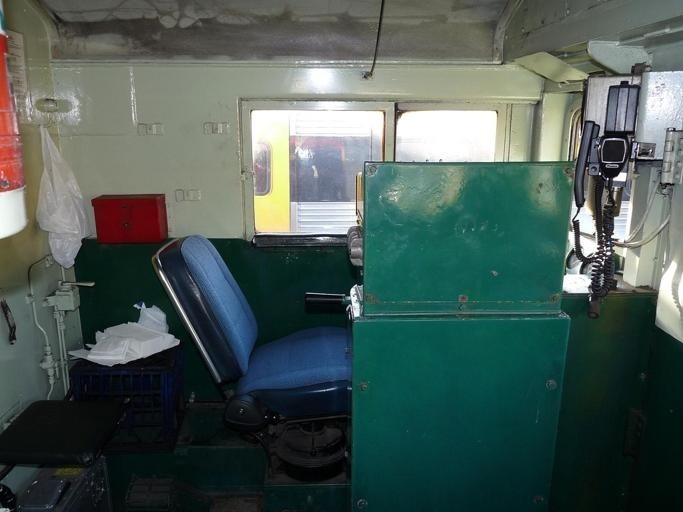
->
[1,398,133,510]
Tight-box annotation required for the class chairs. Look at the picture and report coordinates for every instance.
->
[150,232,353,483]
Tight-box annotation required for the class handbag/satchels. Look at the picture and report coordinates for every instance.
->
[0,396,131,467]
